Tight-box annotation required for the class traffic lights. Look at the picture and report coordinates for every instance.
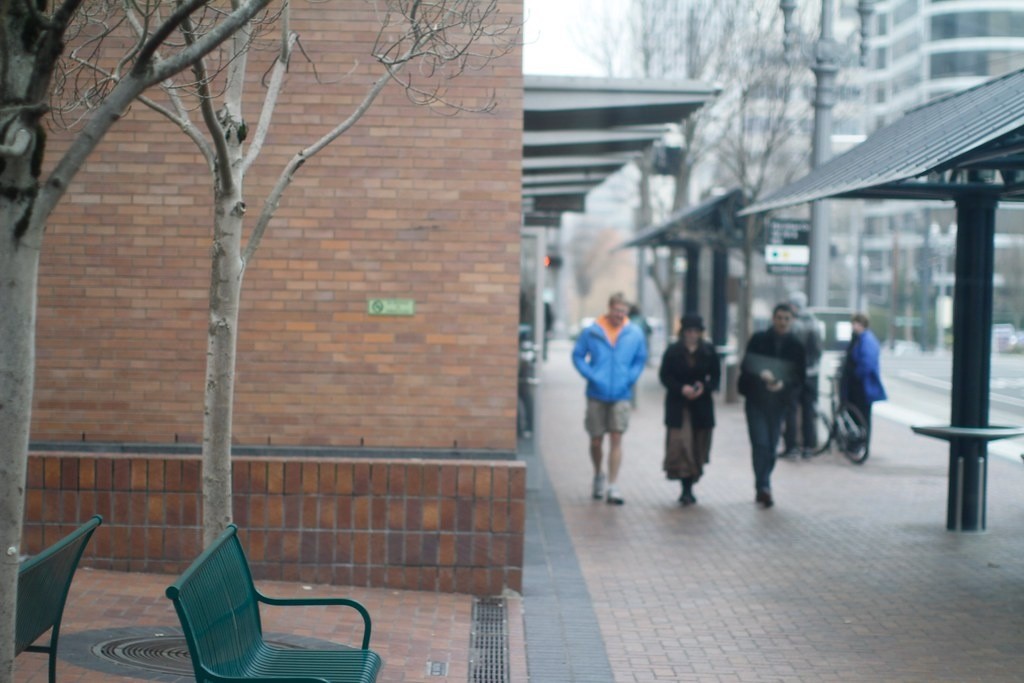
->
[545,255,562,267]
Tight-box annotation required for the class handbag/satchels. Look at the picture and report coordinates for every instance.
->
[738,372,759,396]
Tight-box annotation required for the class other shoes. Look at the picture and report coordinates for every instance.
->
[679,490,696,504]
[605,484,624,505]
[592,476,604,499]
[778,445,813,460]
[756,487,774,507]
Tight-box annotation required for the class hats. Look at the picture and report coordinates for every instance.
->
[676,314,706,336]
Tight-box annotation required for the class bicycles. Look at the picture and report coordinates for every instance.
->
[795,375,869,464]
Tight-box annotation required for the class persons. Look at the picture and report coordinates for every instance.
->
[732,301,809,507]
[569,291,646,505]
[777,290,829,464]
[838,311,887,455]
[656,311,725,505]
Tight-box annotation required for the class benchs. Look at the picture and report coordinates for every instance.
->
[165,523,382,682]
[18,514,104,682]
